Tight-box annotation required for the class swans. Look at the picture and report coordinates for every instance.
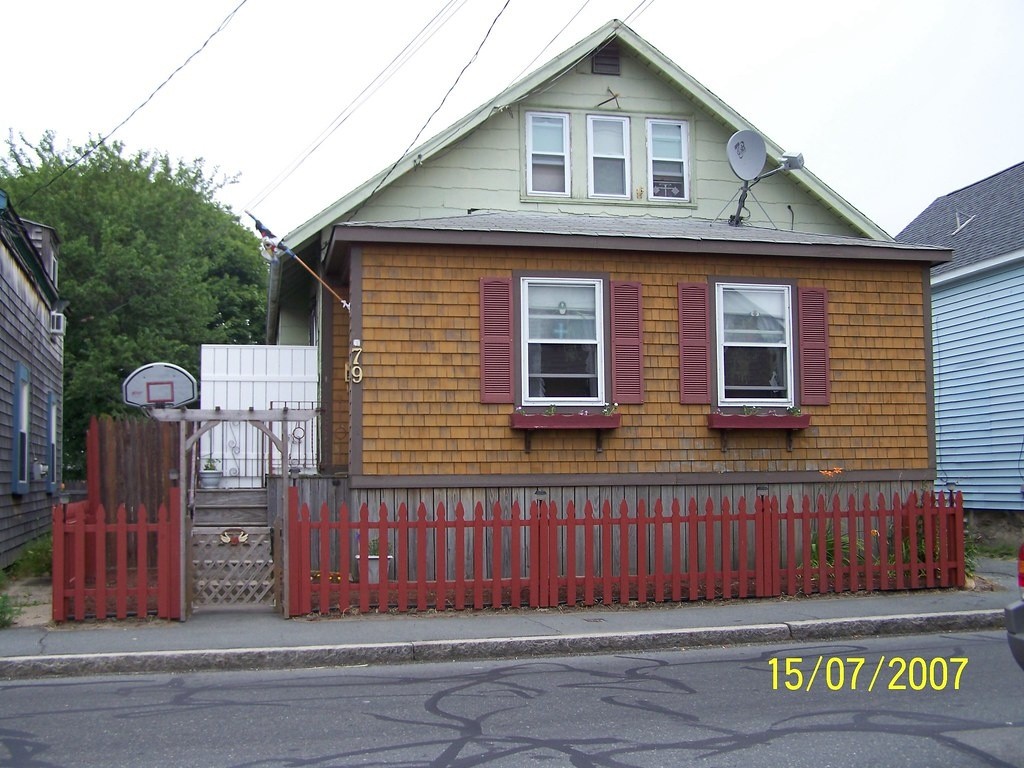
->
[238,531,249,542]
[220,532,230,543]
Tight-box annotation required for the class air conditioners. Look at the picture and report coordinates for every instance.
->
[50,312,66,337]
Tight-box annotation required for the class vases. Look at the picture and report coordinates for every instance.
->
[707,413,811,429]
[508,413,622,428]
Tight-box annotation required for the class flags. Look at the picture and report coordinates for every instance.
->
[255,221,295,262]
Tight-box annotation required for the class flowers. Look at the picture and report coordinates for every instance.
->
[785,403,803,417]
[514,405,527,416]
[743,404,763,417]
[578,409,591,417]
[602,402,620,417]
[714,408,723,415]
[767,409,778,415]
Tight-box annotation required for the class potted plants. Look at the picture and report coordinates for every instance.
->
[355,534,393,584]
[199,454,223,489]
